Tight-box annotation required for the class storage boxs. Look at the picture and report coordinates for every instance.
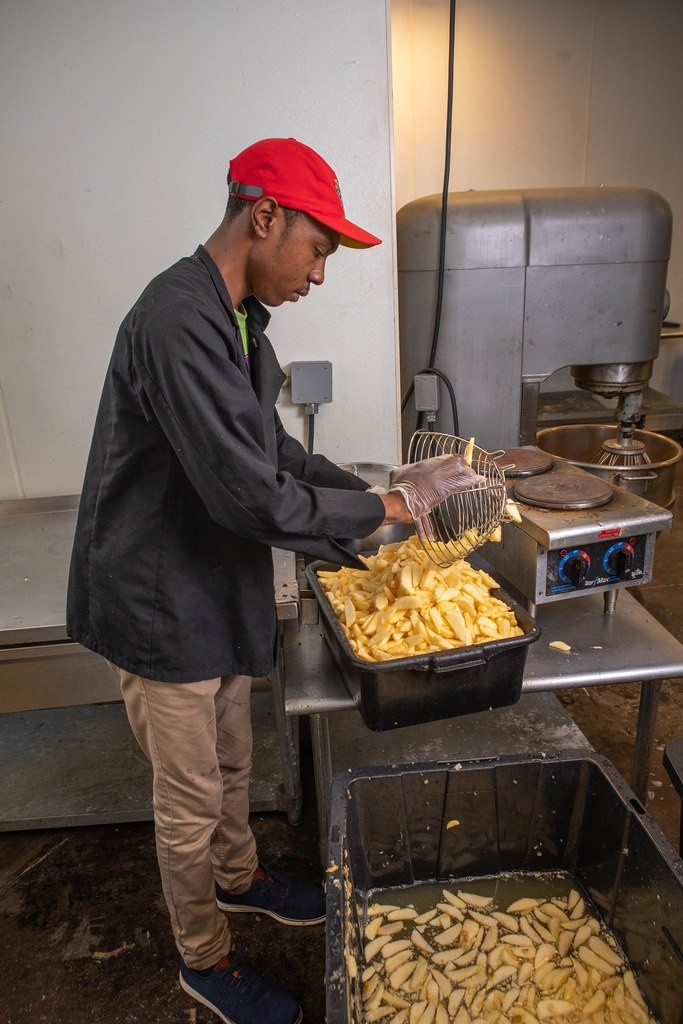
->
[325,750,683,1024]
[304,538,542,734]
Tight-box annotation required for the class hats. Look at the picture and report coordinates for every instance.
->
[227,138,382,249]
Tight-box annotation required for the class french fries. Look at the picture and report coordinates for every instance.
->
[316,437,525,661]
[346,889,657,1024]
[447,820,460,830]
[549,641,572,650]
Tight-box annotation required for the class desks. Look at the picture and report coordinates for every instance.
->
[279,586,683,884]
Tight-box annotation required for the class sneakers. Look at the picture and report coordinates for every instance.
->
[215,863,325,926]
[179,950,303,1024]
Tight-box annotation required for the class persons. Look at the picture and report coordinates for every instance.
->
[64,135,486,1024]
